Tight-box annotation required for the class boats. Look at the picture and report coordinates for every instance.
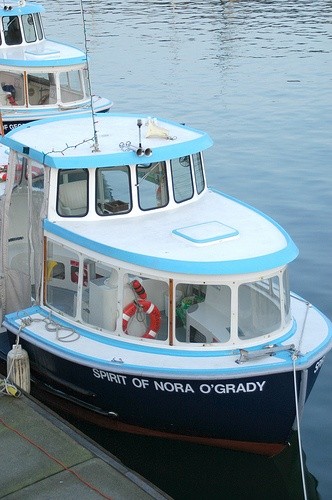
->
[0,1,114,139]
[0,1,332,459]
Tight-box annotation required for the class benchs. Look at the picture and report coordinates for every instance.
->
[185,301,247,343]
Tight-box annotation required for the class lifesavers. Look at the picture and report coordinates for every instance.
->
[0,164,41,181]
[122,300,160,339]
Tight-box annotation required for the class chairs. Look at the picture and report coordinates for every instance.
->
[59,179,88,216]
